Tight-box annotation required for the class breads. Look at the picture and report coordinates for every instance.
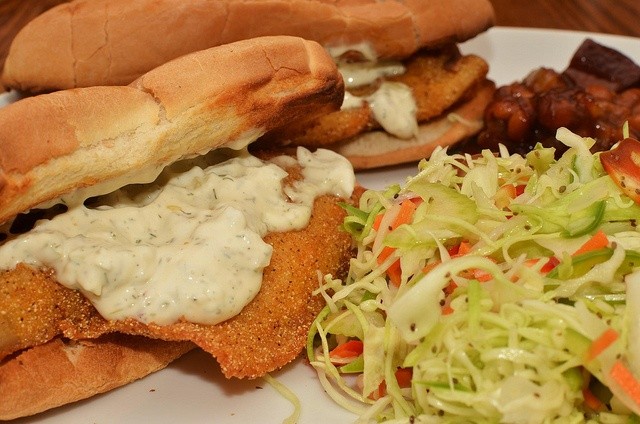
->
[1,0,501,170]
[0,35,367,424]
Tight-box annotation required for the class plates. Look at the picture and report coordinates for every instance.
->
[1,26,639,423]
